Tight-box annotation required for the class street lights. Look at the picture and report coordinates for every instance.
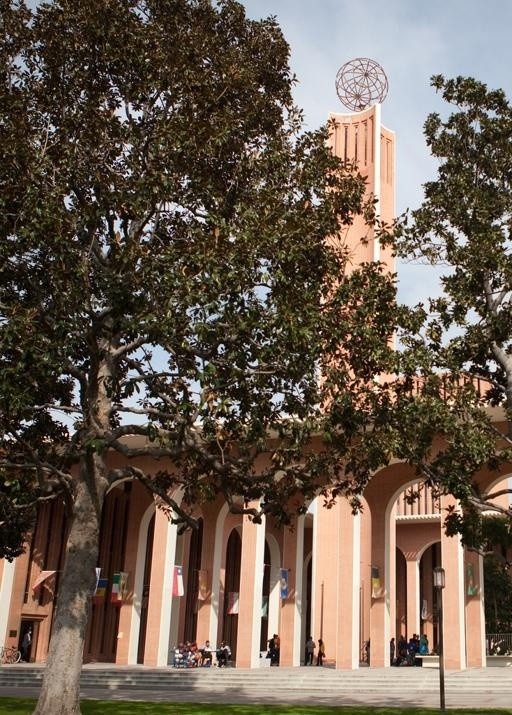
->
[431,565,448,714]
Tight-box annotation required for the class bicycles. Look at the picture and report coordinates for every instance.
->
[0,644,22,665]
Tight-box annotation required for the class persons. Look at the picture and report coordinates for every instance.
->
[259,633,280,667]
[315,639,325,667]
[20,629,34,663]
[489,636,503,656]
[364,637,370,666]
[172,640,232,669]
[389,633,439,667]
[304,636,316,667]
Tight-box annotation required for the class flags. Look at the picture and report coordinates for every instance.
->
[195,569,208,602]
[279,567,289,600]
[226,591,239,616]
[370,566,383,600]
[419,598,429,622]
[467,563,476,597]
[172,564,186,598]
[94,565,131,606]
[30,570,58,591]
[261,595,268,619]
